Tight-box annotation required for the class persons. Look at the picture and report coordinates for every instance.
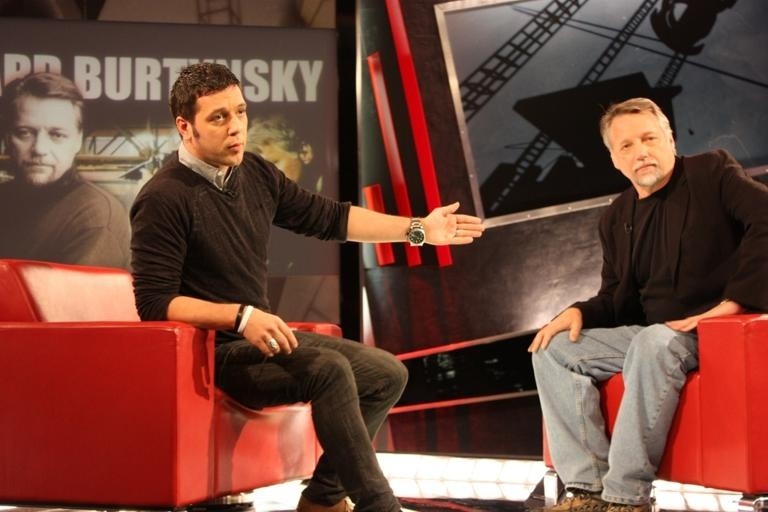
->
[244,113,314,187]
[129,62,486,512]
[0,73,133,273]
[525,97,768,512]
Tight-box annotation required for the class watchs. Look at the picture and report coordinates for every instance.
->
[406,217,426,247]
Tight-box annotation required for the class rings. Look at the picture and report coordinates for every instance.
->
[268,339,277,350]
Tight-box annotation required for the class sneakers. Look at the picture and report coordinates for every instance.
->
[606,501,654,511]
[524,492,608,511]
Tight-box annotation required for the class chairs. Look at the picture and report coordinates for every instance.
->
[0,258,344,512]
[542,310,768,512]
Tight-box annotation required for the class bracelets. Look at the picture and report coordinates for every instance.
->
[233,304,246,334]
[237,305,254,336]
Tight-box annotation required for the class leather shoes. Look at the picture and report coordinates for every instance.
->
[296,493,353,512]
